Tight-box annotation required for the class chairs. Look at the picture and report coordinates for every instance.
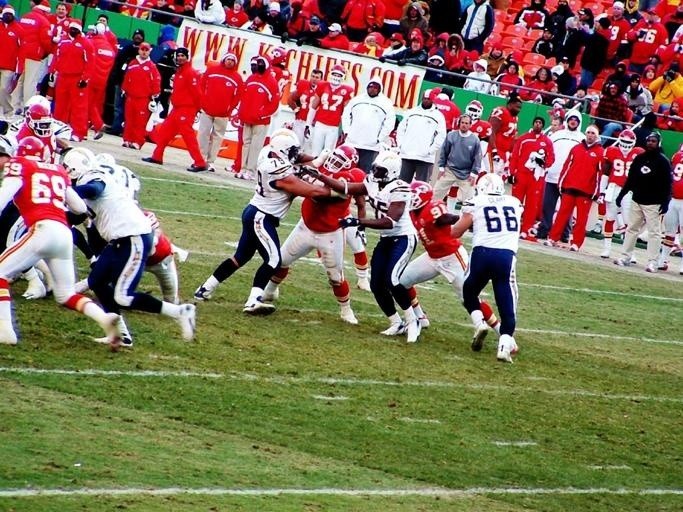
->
[543,57,557,69]
[493,8,506,21]
[489,33,502,45]
[501,25,528,39]
[519,41,535,51]
[583,2,604,16]
[501,36,524,49]
[501,48,522,62]
[523,53,546,67]
[523,29,544,42]
[568,0,583,12]
[597,68,616,80]
[522,64,541,77]
[591,79,607,91]
[492,22,504,34]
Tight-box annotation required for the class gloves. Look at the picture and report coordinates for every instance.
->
[508,176,516,184]
[339,218,359,228]
[535,157,544,167]
[47,73,56,88]
[597,196,604,204]
[304,126,311,139]
[338,133,344,144]
[435,213,459,228]
[303,164,321,179]
[331,189,349,200]
[615,190,624,208]
[658,201,669,215]
[313,149,330,167]
[79,80,87,87]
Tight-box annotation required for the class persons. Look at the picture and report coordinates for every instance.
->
[287,0,325,21]
[551,66,563,83]
[194,0,226,25]
[478,41,504,81]
[494,49,523,81]
[420,1,429,20]
[624,75,656,148]
[225,57,258,172]
[15,106,61,164]
[0,137,124,352]
[116,0,152,20]
[297,152,421,343]
[398,1,427,37]
[241,13,272,35]
[84,22,115,140]
[355,31,385,59]
[658,142,683,276]
[341,0,374,42]
[425,50,452,85]
[533,110,586,249]
[430,88,461,134]
[266,3,286,36]
[0,5,26,120]
[379,36,427,66]
[455,50,478,90]
[264,144,365,324]
[407,30,422,45]
[429,1,461,35]
[561,8,595,51]
[443,34,466,83]
[508,117,556,239]
[62,146,197,345]
[447,101,491,211]
[146,1,179,25]
[120,42,161,150]
[531,24,564,66]
[620,59,632,80]
[374,0,413,37]
[0,0,19,22]
[250,0,267,18]
[194,128,350,318]
[460,0,473,20]
[344,209,372,292]
[579,17,612,89]
[653,1,683,39]
[317,24,350,52]
[451,173,524,363]
[490,61,523,97]
[233,55,280,180]
[649,62,683,112]
[429,33,450,65]
[264,0,290,24]
[603,63,629,98]
[551,84,590,115]
[560,18,588,68]
[595,80,628,144]
[433,115,482,205]
[651,35,682,80]
[224,0,248,27]
[197,53,243,172]
[608,2,632,63]
[382,33,406,59]
[46,3,72,102]
[639,65,657,99]
[12,0,52,115]
[148,0,176,10]
[512,1,549,30]
[459,0,495,50]
[177,0,195,29]
[543,125,604,252]
[657,98,682,131]
[671,25,683,44]
[614,212,629,234]
[463,58,492,97]
[50,18,96,143]
[98,14,118,57]
[589,13,607,37]
[597,129,646,263]
[489,98,522,183]
[623,0,645,29]
[142,48,208,172]
[287,68,322,152]
[519,67,557,106]
[341,75,396,179]
[546,1,574,46]
[268,48,291,105]
[281,16,324,46]
[149,25,177,118]
[305,65,354,159]
[613,131,672,272]
[396,89,446,184]
[104,28,145,135]
[555,55,577,97]
[624,8,669,75]
[399,181,518,354]
[590,123,622,233]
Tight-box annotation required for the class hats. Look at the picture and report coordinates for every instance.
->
[585,124,599,138]
[34,0,51,13]
[390,33,403,42]
[630,74,640,83]
[2,4,15,15]
[176,48,188,59]
[309,16,319,25]
[328,22,342,32]
[69,19,83,33]
[139,42,150,50]
[646,131,661,140]
[613,1,625,9]
[595,18,610,29]
[422,89,433,99]
[533,116,545,128]
[647,8,656,14]
[132,28,144,40]
[547,108,565,119]
[269,2,280,12]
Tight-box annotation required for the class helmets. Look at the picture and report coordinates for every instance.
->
[465,100,483,120]
[369,151,402,182]
[270,128,301,162]
[15,135,46,162]
[477,172,505,195]
[406,181,433,212]
[96,153,116,174]
[323,145,359,173]
[330,64,346,85]
[61,147,98,179]
[619,130,637,151]
[23,95,52,138]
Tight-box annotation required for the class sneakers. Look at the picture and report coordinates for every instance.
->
[406,318,421,343]
[380,322,405,336]
[208,162,216,171]
[498,332,518,353]
[659,260,668,270]
[177,303,196,343]
[544,239,555,247]
[569,243,578,252]
[240,174,254,180]
[193,283,212,301]
[186,164,207,172]
[122,141,129,147]
[0,329,18,345]
[471,323,488,352]
[645,259,659,273]
[243,298,276,316]
[631,257,637,263]
[224,166,234,172]
[94,332,133,347]
[601,252,610,258]
[131,142,139,150]
[262,287,279,300]
[357,279,371,292]
[519,231,527,239]
[102,313,121,352]
[613,254,630,267]
[234,170,245,178]
[406,314,429,332]
[496,344,513,363]
[341,306,358,324]
[93,124,106,140]
[21,278,45,300]
[142,157,163,165]
